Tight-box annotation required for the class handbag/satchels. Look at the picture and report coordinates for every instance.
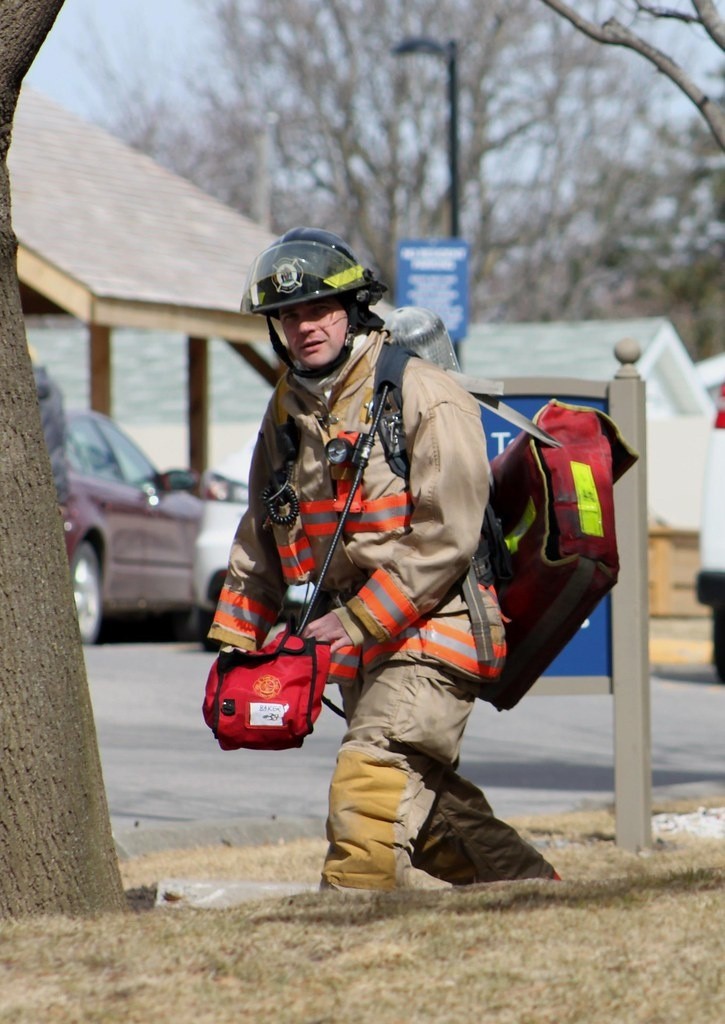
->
[203,633,333,750]
[473,399,638,712]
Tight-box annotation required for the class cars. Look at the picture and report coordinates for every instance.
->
[161,467,249,642]
[58,410,206,645]
[695,365,725,685]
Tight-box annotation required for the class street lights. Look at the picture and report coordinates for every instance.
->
[390,36,462,369]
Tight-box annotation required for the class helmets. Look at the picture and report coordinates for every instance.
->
[250,226,388,319]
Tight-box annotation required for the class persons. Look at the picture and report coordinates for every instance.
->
[205,228,561,892]
